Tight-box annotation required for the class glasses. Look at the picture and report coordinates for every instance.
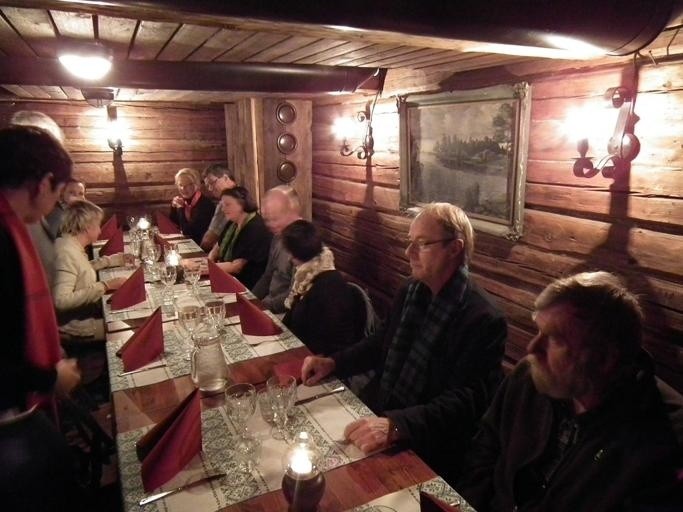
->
[405,237,447,249]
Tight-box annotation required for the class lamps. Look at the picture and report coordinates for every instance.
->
[330,89,380,158]
[574,51,646,177]
[57,43,113,81]
[81,88,114,109]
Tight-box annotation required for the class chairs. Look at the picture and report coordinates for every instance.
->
[52,394,115,469]
[654,374,683,454]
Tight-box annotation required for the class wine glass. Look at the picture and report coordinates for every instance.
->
[121,211,232,394]
[223,382,262,454]
[264,374,302,442]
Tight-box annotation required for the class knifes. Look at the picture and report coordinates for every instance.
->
[137,472,227,507]
[293,386,346,407]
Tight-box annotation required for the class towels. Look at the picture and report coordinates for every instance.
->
[155,211,181,233]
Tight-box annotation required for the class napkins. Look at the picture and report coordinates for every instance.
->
[208,258,247,294]
[98,230,124,257]
[136,386,203,494]
[261,353,325,389]
[150,231,174,262]
[106,264,148,311]
[235,291,283,337]
[115,305,165,373]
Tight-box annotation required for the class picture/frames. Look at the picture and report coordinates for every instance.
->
[396,81,532,241]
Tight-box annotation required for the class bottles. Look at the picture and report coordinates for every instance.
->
[280,431,326,512]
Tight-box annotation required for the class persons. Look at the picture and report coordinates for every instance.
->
[251,185,306,322]
[301,202,508,470]
[284,218,356,358]
[455,268,682,512]
[198,163,236,253]
[14,110,134,408]
[169,169,214,245]
[1,125,82,512]
[206,186,271,290]
